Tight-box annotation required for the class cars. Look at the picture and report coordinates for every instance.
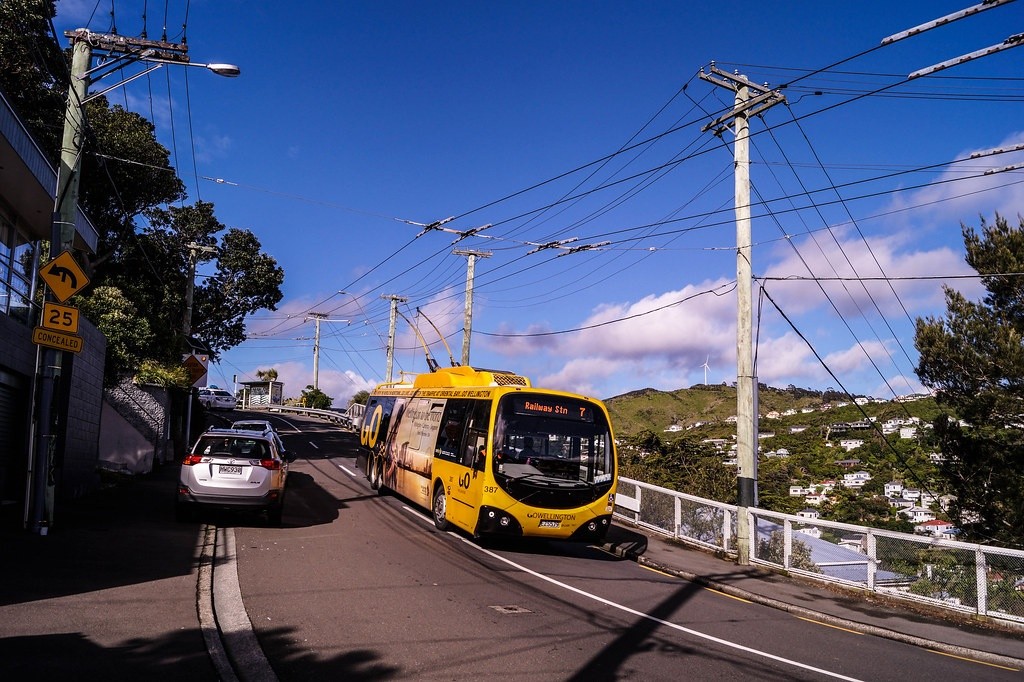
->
[198,389,237,412]
[179,420,298,523]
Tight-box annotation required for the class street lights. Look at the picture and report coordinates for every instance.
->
[24,28,241,536]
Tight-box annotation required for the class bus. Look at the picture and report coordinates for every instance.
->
[355,366,617,541]
